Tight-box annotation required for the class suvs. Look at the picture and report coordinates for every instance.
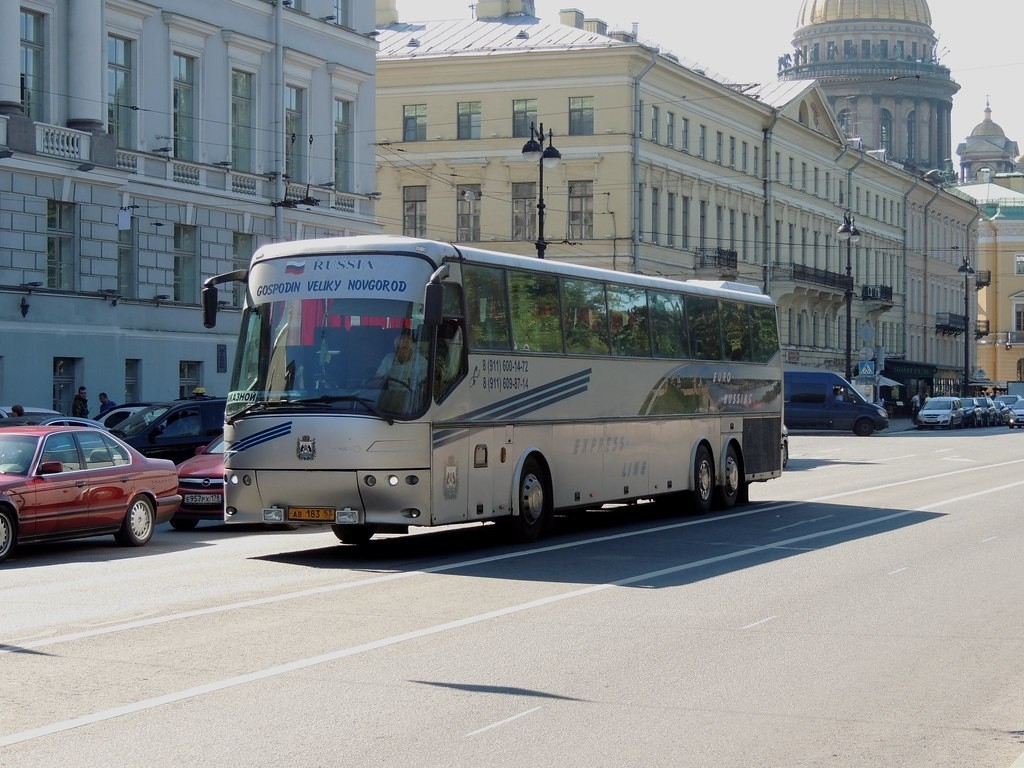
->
[972,395,998,427]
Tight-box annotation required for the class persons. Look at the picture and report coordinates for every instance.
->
[911,392,921,425]
[924,393,932,403]
[99,392,117,413]
[836,389,849,402]
[374,334,428,391]
[72,386,89,419]
[12,404,25,417]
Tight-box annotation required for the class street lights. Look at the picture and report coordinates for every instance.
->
[957,255,975,398]
[836,211,861,385]
[522,120,563,260]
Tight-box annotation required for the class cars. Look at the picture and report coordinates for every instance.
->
[958,397,984,428]
[1008,399,1024,429]
[0,387,227,466]
[917,396,966,431]
[992,394,1022,426]
[168,431,224,530]
[0,425,183,565]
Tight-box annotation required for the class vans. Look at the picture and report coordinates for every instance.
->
[781,369,889,437]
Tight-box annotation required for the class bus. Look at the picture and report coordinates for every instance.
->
[200,234,782,545]
